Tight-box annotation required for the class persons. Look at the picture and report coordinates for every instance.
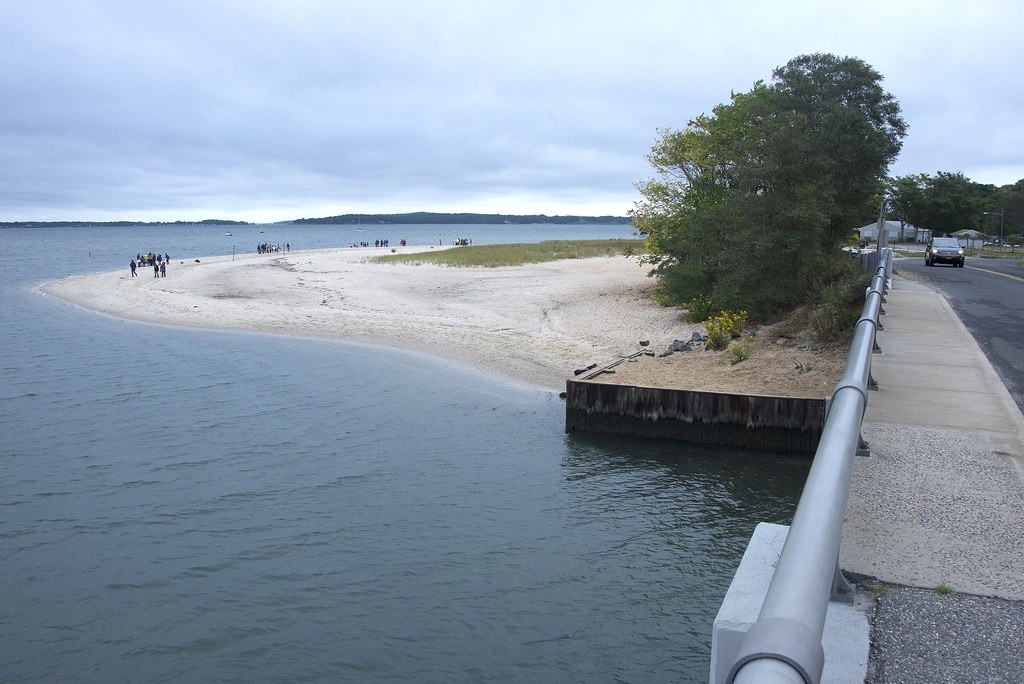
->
[258,243,289,254]
[455,238,472,245]
[351,241,368,248]
[375,239,388,247]
[130,252,170,277]
[401,239,406,246]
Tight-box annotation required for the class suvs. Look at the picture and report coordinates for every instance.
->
[925,236,966,267]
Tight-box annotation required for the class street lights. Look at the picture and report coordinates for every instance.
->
[983,206,1003,247]
